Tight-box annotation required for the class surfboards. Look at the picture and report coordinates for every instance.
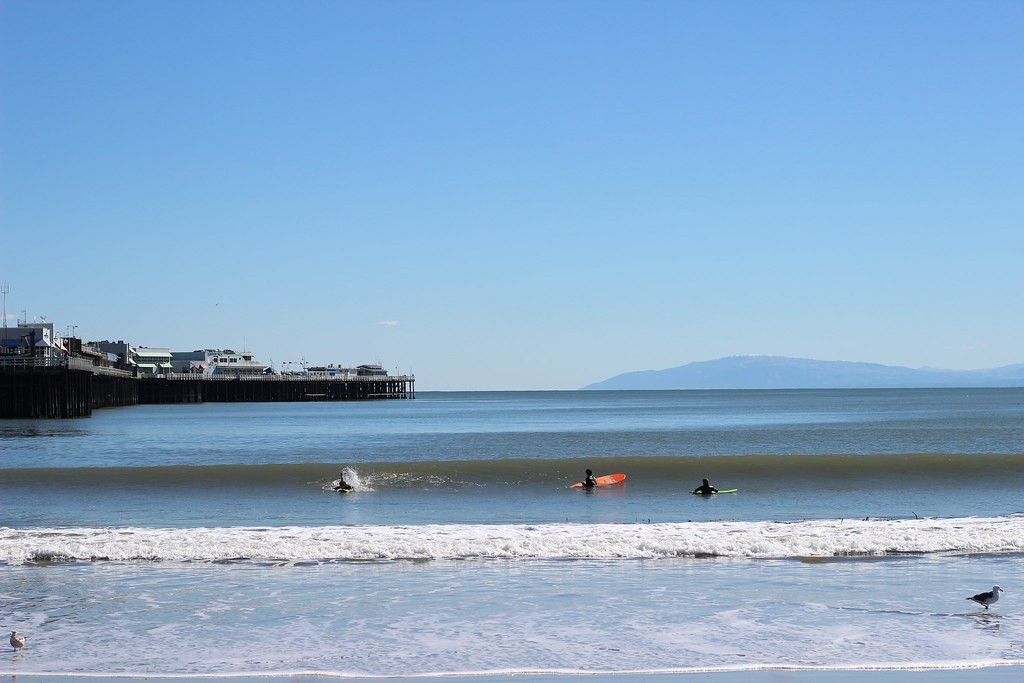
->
[690,488,738,494]
[337,488,355,493]
[570,473,626,490]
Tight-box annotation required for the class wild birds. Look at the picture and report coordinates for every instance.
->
[965,585,1004,609]
[9,630,28,652]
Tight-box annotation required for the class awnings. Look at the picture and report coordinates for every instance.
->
[55,342,69,352]
[137,363,157,367]
[160,363,172,368]
[49,342,61,353]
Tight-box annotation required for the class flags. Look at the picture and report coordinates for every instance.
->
[282,360,308,365]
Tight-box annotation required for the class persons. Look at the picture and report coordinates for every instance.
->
[695,478,718,494]
[581,467,598,487]
[332,472,352,489]
